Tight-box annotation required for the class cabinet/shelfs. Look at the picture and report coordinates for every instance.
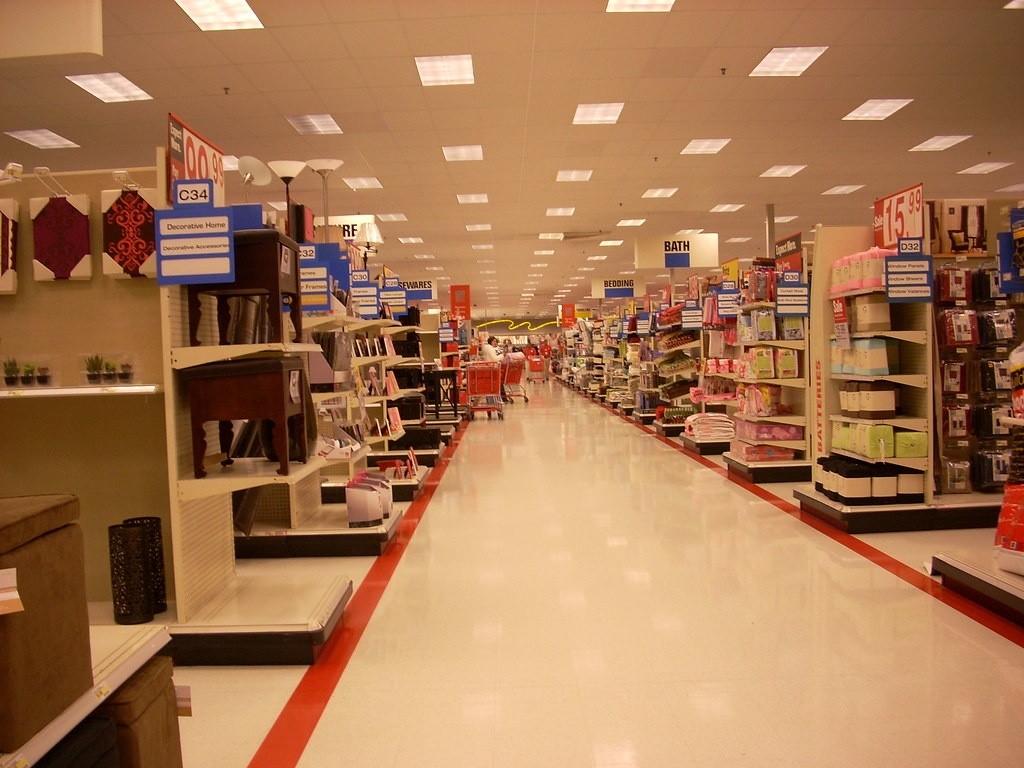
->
[543,223,1024,619]
[0,146,509,666]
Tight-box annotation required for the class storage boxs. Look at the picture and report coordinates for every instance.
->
[0,493,98,756]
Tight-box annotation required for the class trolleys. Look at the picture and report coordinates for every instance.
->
[463,360,504,421]
[501,356,529,405]
[526,354,546,384]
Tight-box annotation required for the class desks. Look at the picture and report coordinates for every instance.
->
[0,624,184,768]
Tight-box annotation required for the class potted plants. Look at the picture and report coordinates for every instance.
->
[3,358,20,386]
[36,367,52,386]
[21,363,35,386]
[118,363,134,383]
[102,361,118,385]
[84,354,103,386]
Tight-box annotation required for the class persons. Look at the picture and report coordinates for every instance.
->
[512,339,552,381]
[482,336,509,404]
